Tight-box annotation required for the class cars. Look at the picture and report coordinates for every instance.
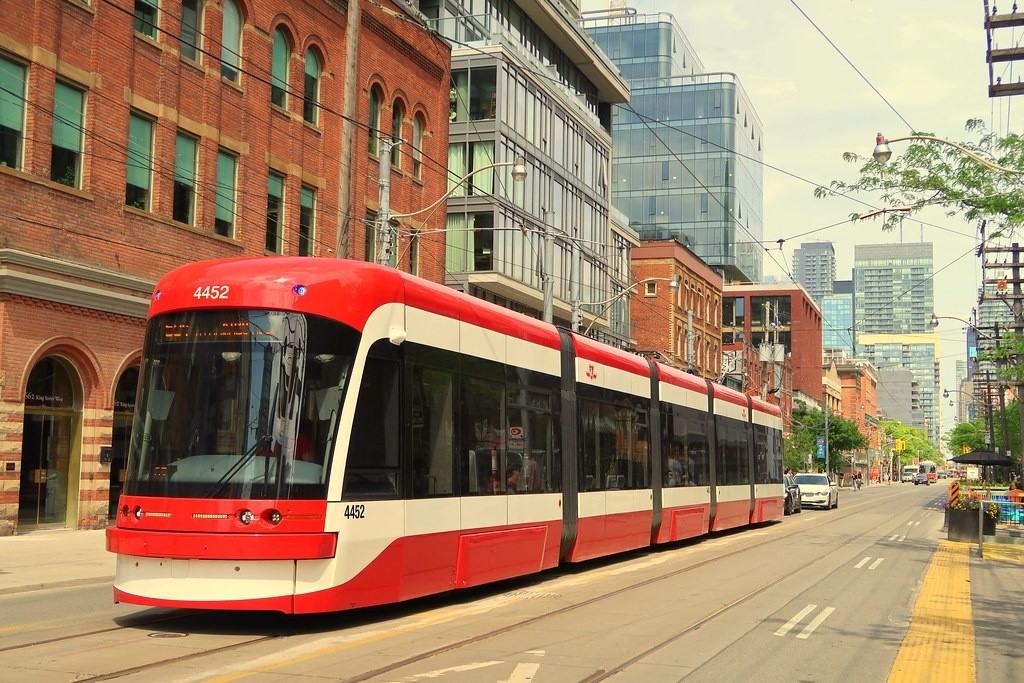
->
[793,472,839,508]
[784,472,802,516]
[900,459,957,486]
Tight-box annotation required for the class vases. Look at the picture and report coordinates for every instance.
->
[945,509,996,543]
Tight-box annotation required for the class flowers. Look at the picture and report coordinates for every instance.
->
[943,492,1001,524]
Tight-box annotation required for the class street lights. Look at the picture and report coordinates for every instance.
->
[572,241,680,333]
[379,137,527,268]
[872,132,1024,174]
[930,313,1010,456]
[943,369,998,482]
[825,393,865,476]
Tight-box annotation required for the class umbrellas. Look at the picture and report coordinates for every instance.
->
[949,446,1011,479]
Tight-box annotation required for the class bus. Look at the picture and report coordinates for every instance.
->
[105,255,785,636]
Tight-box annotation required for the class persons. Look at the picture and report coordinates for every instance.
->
[1011,473,1018,482]
[888,473,891,485]
[785,468,793,475]
[852,471,862,491]
[839,472,845,488]
[676,448,694,478]
[257,411,314,464]
[508,448,538,493]
